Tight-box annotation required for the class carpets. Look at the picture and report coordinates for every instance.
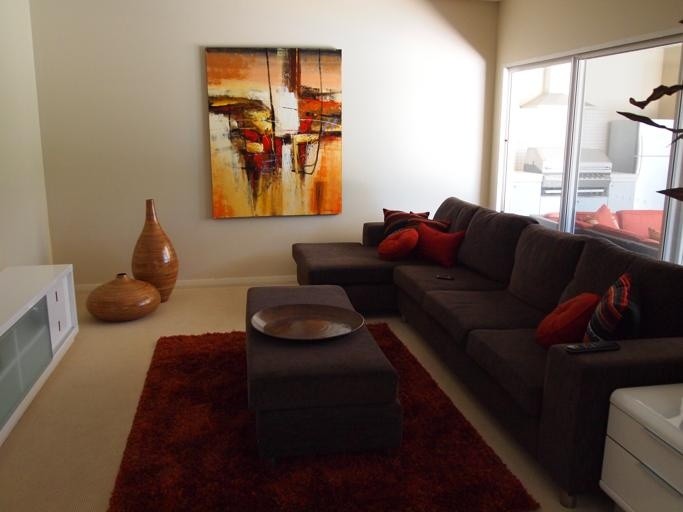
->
[104,319,542,512]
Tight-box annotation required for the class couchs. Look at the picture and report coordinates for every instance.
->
[288,196,681,508]
[526,202,665,258]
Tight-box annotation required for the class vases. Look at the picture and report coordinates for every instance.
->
[130,199,179,303]
[82,272,161,326]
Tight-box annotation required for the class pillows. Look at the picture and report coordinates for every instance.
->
[412,220,465,270]
[375,207,429,246]
[583,203,621,230]
[404,209,453,235]
[644,225,662,242]
[579,268,633,345]
[531,291,603,350]
[376,226,420,260]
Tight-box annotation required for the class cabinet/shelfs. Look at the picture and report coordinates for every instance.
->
[597,379,682,511]
[0,263,80,450]
